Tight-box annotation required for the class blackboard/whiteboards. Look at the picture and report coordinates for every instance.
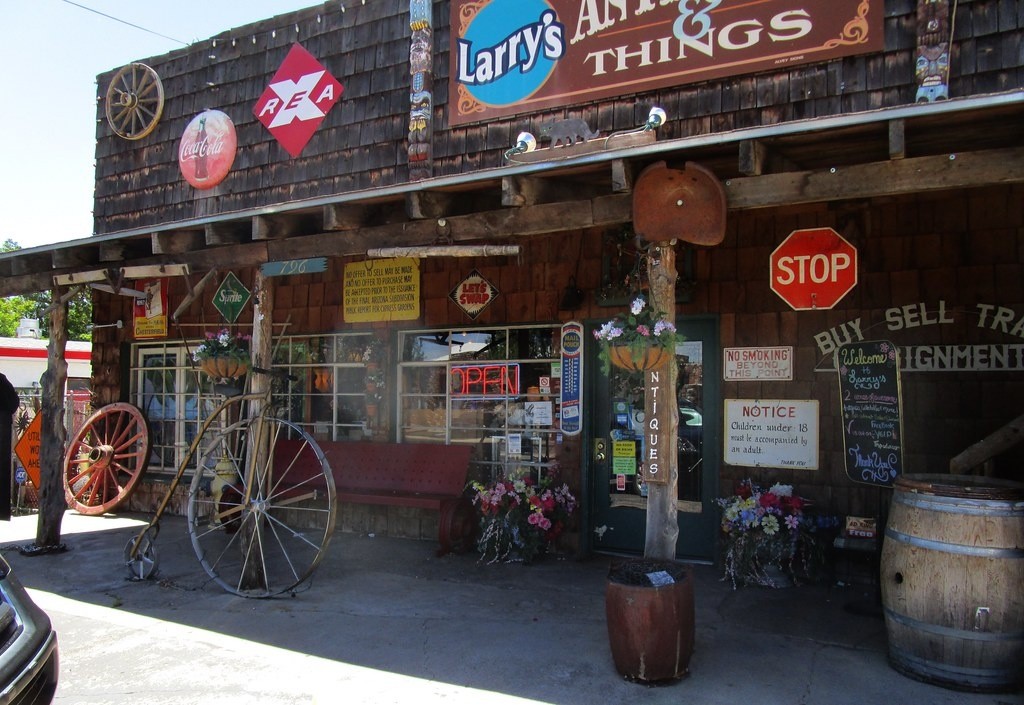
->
[838,340,906,487]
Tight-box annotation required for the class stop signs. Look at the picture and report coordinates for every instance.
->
[769,226,857,311]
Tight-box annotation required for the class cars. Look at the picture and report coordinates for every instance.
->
[611,397,703,501]
[0,553,59,705]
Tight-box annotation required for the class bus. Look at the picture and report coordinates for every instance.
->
[402,359,561,427]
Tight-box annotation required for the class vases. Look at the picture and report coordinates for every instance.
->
[200,356,250,385]
[604,558,695,689]
[607,346,674,372]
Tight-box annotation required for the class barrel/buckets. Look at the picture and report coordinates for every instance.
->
[878,474,1024,695]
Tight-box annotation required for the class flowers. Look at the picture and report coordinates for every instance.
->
[460,470,536,567]
[527,483,577,557]
[193,328,252,371]
[709,478,815,587]
[592,294,686,379]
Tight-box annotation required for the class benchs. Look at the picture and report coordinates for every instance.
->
[219,439,480,554]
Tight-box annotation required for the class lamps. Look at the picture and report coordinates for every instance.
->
[504,132,537,164]
[604,107,666,151]
[85,320,123,331]
